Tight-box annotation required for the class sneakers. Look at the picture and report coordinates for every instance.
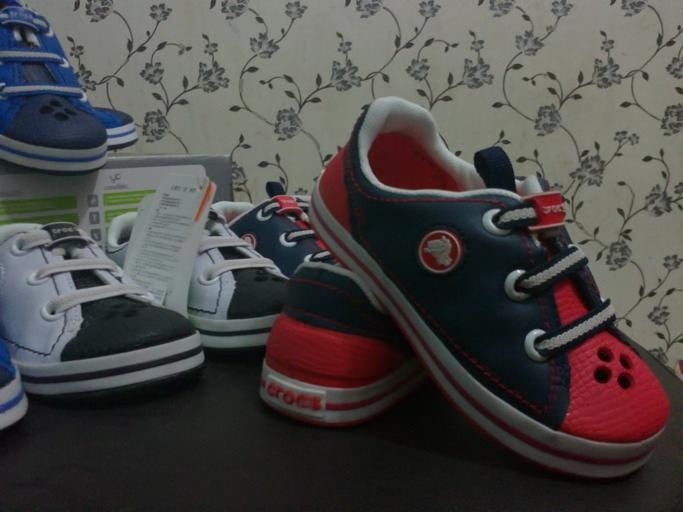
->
[212,180,347,280]
[0,219,206,398]
[307,96,670,485]
[0,0,108,174]
[92,103,138,149]
[260,249,432,426]
[0,334,29,430]
[105,201,289,347]
